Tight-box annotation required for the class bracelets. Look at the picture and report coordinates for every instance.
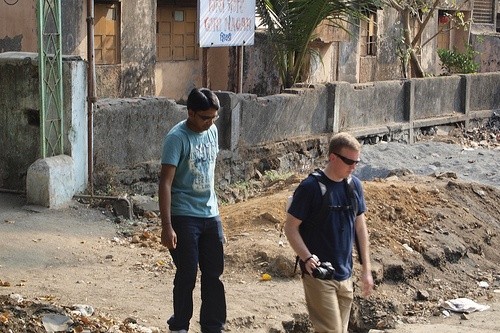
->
[304,256,313,264]
[161,223,172,226]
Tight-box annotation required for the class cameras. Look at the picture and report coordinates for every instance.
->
[311,261,335,280]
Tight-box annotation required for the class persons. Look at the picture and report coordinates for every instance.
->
[159,88,227,333]
[284,132,375,333]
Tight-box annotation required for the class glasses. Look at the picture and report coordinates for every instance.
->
[330,152,360,165]
[194,112,218,121]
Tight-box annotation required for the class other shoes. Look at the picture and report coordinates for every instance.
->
[171,329,188,333]
